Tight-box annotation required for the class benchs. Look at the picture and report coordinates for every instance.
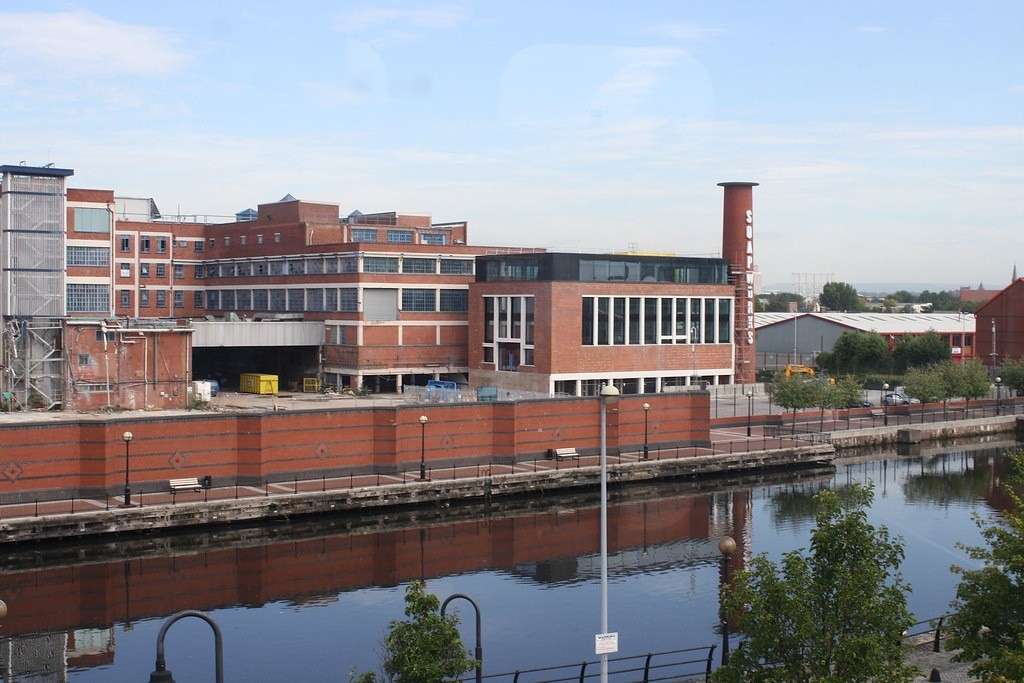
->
[555,448,581,461]
[168,477,202,494]
[870,408,886,418]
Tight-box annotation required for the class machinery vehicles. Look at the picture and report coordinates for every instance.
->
[786,364,836,387]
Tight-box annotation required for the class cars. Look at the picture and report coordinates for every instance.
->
[842,397,874,408]
[881,392,922,405]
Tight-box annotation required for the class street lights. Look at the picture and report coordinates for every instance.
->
[121,559,135,631]
[121,431,137,508]
[432,592,482,683]
[995,447,1001,489]
[746,391,753,436]
[641,502,649,558]
[418,415,428,481]
[884,383,890,426]
[643,403,650,459]
[600,377,622,681]
[882,459,887,499]
[717,537,738,666]
[419,529,428,590]
[995,376,1002,416]
